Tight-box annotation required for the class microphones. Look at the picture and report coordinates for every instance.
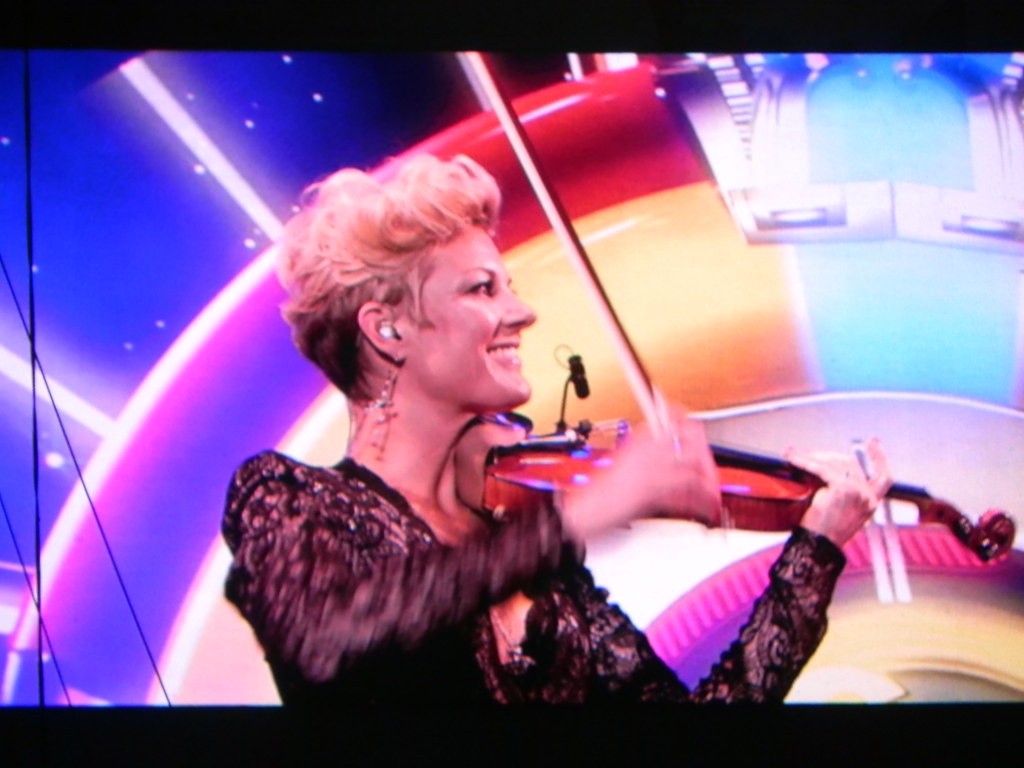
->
[569,355,591,399]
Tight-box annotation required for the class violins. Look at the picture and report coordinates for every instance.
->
[454,404,1018,563]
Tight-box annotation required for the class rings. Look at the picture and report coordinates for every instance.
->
[673,437,683,465]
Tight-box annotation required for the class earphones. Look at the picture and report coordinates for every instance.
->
[377,326,395,340]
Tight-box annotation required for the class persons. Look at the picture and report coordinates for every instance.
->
[220,155,892,706]
